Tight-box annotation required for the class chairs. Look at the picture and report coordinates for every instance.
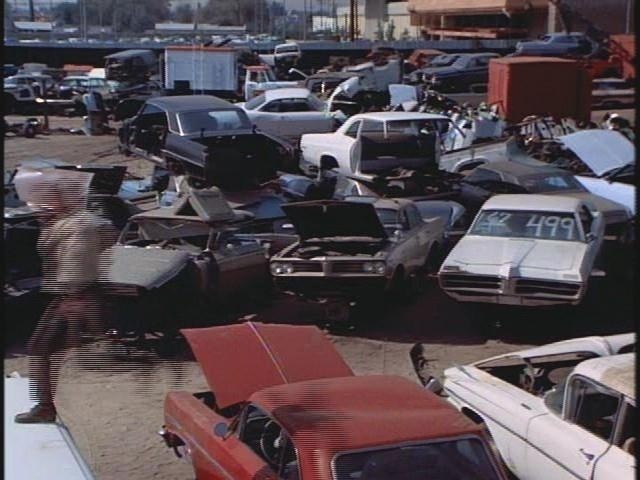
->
[14,403,57,423]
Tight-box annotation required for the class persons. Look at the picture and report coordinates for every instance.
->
[422,71,444,104]
[611,120,634,144]
[600,112,619,130]
[13,177,187,425]
[88,90,110,124]
[327,73,370,112]
[77,88,97,129]
[325,57,349,73]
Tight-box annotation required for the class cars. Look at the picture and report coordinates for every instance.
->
[1,33,640,227]
[437,331,639,479]
[433,189,606,314]
[92,186,271,321]
[266,194,469,309]
[158,320,511,479]
